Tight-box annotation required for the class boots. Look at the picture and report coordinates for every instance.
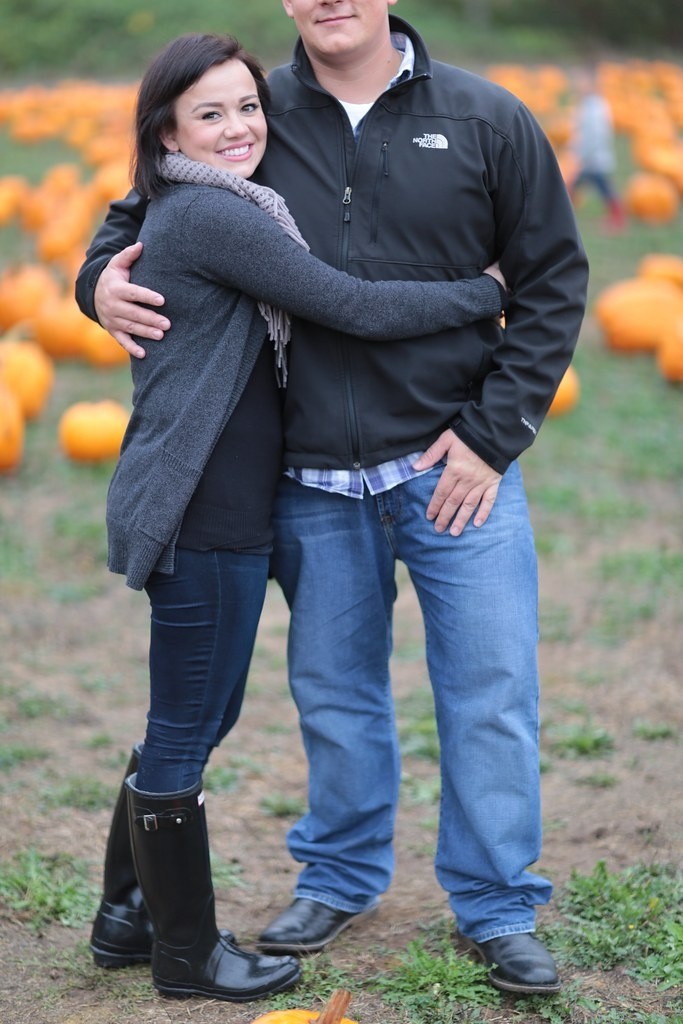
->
[90,746,301,1001]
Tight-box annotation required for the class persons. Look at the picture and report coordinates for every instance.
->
[76,0,593,995]
[91,33,508,1001]
[568,63,624,231]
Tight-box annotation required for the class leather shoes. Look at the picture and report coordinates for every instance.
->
[255,899,382,951]
[457,925,562,993]
